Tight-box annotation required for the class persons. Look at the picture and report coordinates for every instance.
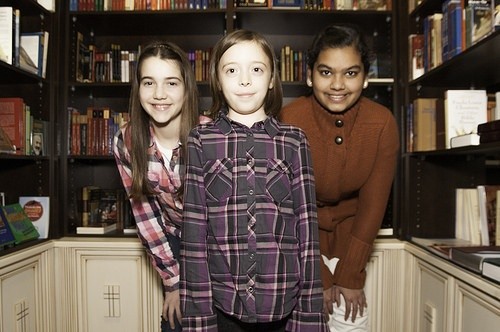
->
[280,25,397,332]
[180,30,323,332]
[114,39,213,332]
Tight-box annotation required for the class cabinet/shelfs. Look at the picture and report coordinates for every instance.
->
[404,237,500,332]
[62,0,399,239]
[55,236,404,332]
[0,239,54,332]
[0,0,62,258]
[399,0,500,286]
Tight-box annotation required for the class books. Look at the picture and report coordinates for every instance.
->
[0,0,500,286]
